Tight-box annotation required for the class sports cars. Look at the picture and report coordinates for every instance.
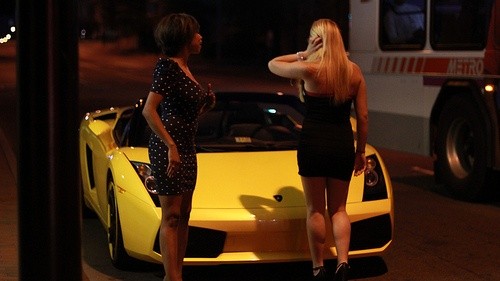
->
[79,87,395,268]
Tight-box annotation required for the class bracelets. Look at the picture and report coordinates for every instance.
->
[297,52,304,60]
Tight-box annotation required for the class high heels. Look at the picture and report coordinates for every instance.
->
[310,265,328,281]
[333,262,350,281]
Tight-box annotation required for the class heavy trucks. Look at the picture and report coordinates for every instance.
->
[373,1,500,197]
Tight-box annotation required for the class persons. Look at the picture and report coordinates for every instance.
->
[142,13,216,281]
[267,18,368,281]
